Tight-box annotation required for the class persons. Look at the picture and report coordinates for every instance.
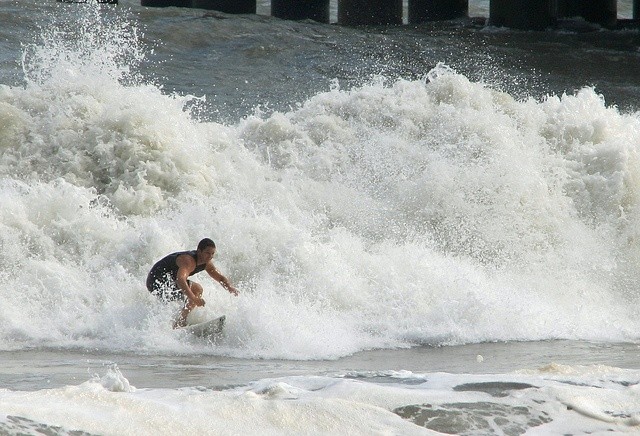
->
[146,238,240,329]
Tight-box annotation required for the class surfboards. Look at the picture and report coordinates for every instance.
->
[174,316,227,336]
